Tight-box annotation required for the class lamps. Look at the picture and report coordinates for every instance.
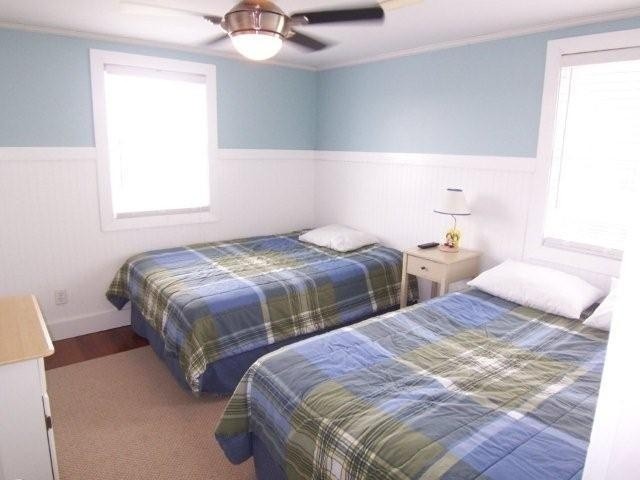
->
[432,189,473,253]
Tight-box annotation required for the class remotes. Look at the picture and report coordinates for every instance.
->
[418,242,439,249]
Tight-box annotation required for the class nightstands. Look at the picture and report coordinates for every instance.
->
[399,245,480,308]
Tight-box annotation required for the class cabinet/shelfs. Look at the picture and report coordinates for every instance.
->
[0,291,60,480]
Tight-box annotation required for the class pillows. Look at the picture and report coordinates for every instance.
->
[297,223,380,254]
[469,256,622,330]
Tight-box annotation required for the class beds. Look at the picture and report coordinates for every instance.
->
[104,229,420,398]
[212,288,613,480]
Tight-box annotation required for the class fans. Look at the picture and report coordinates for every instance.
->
[117,1,384,52]
